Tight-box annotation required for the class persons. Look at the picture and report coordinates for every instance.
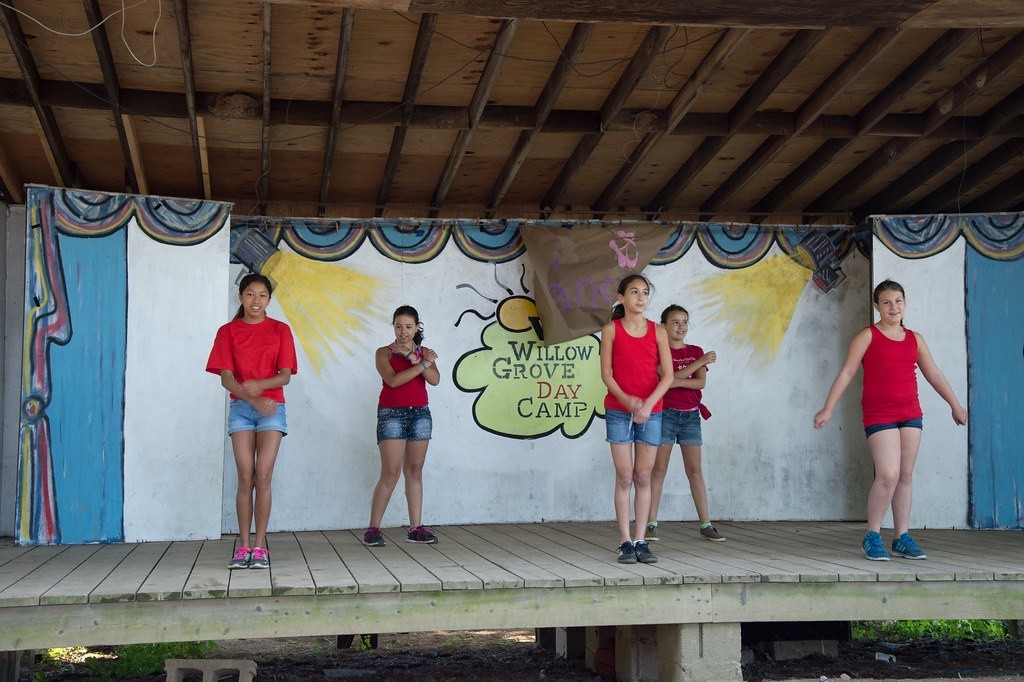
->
[362,305,440,547]
[814,278,968,561]
[205,273,298,569]
[644,304,726,542]
[600,272,674,564]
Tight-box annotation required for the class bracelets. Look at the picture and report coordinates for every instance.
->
[403,347,421,365]
[419,358,434,369]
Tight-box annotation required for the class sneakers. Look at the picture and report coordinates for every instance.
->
[406,525,437,544]
[645,525,659,541]
[700,526,726,542]
[615,541,637,563]
[363,527,386,546]
[890,532,927,559]
[633,539,657,562]
[861,530,890,560]
[248,547,270,569]
[228,545,252,568]
